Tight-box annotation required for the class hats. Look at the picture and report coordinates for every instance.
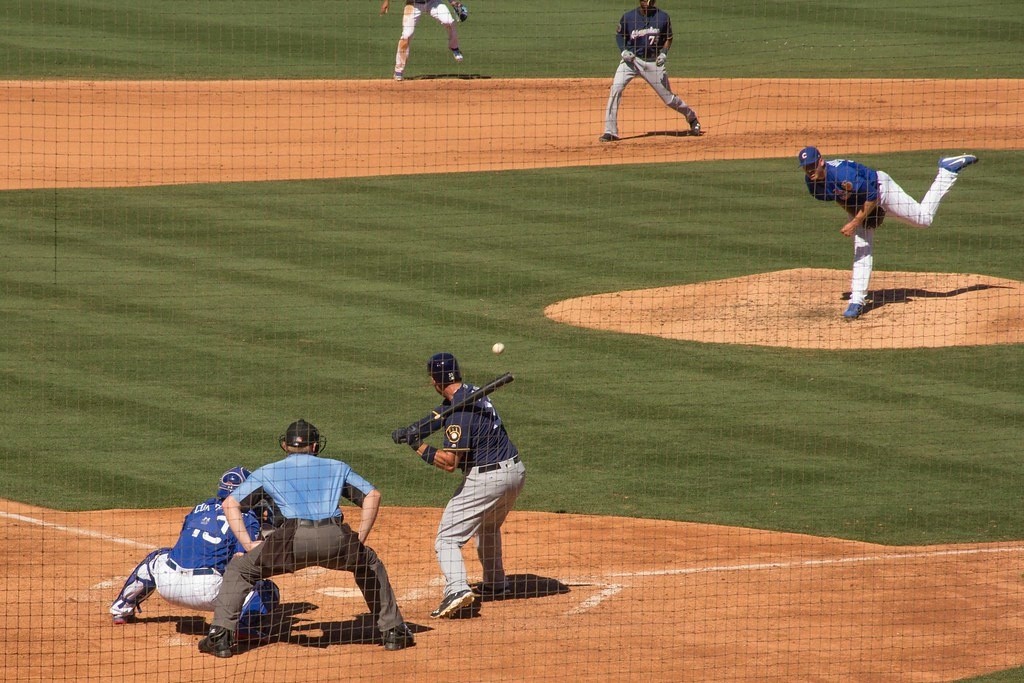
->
[798,146,821,167]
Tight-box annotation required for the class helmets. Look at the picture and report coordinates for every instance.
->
[216,466,253,499]
[285,418,319,447]
[427,353,462,389]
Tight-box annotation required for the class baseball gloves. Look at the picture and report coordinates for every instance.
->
[862,207,885,229]
[253,504,284,526]
[451,1,468,22]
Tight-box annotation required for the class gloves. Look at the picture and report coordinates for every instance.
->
[392,427,408,444]
[621,50,635,63]
[406,426,424,452]
[656,53,667,67]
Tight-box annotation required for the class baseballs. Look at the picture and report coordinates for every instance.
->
[492,343,505,354]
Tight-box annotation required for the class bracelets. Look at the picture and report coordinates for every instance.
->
[420,444,438,465]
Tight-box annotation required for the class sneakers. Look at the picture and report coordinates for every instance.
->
[394,71,403,81]
[383,625,415,649]
[845,302,868,318]
[198,625,233,658]
[690,118,701,134]
[450,48,463,61]
[600,133,618,142]
[472,584,511,596]
[938,154,979,173]
[235,622,268,644]
[430,590,475,619]
[113,613,135,624]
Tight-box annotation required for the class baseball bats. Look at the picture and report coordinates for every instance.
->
[419,371,515,430]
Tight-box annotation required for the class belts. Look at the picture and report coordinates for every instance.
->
[166,559,214,576]
[467,456,520,473]
[285,517,344,527]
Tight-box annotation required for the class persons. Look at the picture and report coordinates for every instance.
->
[379,0,468,81]
[109,465,280,639]
[798,147,979,318]
[598,0,701,141]
[199,419,414,660]
[392,353,527,618]
